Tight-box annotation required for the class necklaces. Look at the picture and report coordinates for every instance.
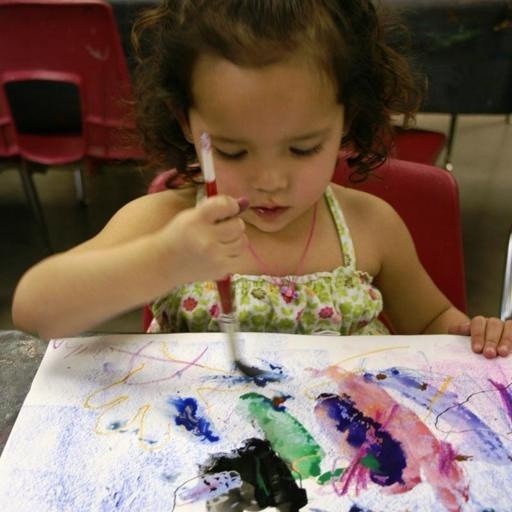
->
[239,200,318,303]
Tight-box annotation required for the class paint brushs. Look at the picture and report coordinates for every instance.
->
[201,132,263,365]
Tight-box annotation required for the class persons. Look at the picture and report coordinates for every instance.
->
[12,1,510,360]
